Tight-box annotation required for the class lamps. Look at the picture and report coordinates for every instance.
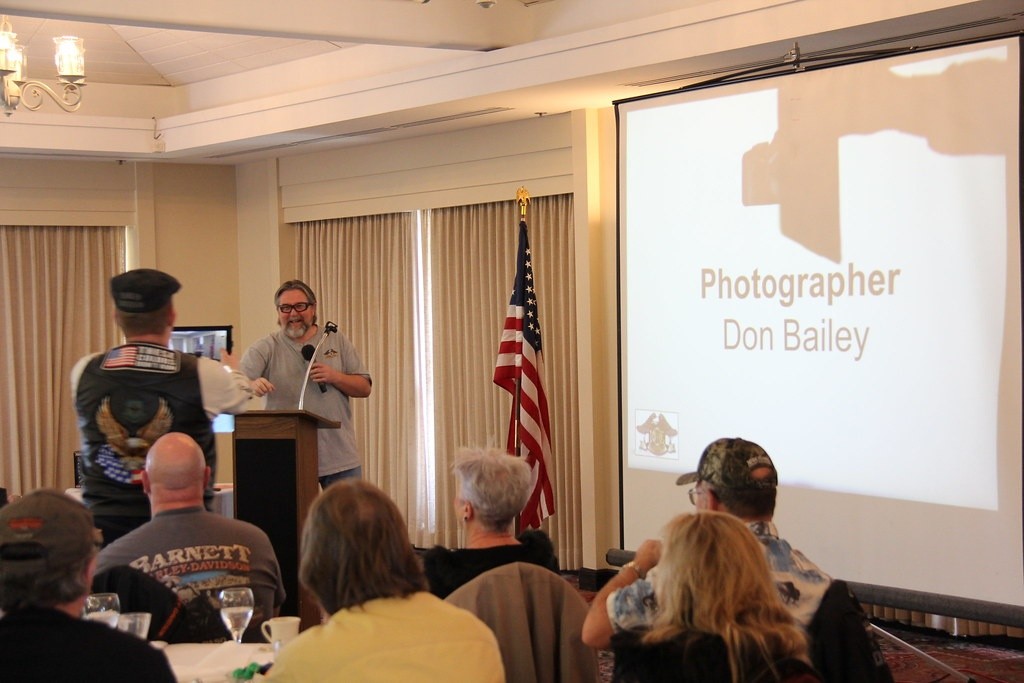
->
[0,14,87,117]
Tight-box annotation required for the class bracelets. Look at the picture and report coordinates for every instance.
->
[623,561,644,579]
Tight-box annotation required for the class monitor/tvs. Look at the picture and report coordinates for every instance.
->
[168,325,233,361]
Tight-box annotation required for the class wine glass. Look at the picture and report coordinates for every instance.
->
[220,587,254,645]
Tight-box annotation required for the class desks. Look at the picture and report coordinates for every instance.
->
[151,641,275,683]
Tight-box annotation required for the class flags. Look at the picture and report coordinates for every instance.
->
[495,220,556,540]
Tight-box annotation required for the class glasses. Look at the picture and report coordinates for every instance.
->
[277,302,311,313]
[688,489,708,505]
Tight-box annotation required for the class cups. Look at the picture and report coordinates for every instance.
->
[81,592,120,629]
[118,613,152,640]
[261,617,301,650]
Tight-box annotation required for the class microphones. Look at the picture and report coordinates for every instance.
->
[301,344,327,394]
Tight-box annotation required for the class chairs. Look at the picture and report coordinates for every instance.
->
[444,561,602,683]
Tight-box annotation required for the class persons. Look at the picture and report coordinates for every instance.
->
[262,479,506,683]
[69,269,249,548]
[418,446,561,599]
[608,512,815,683]
[96,433,286,644]
[0,488,178,683]
[239,280,372,492]
[581,439,833,649]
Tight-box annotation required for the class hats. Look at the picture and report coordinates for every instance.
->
[675,437,778,495]
[0,488,94,575]
[110,269,182,313]
